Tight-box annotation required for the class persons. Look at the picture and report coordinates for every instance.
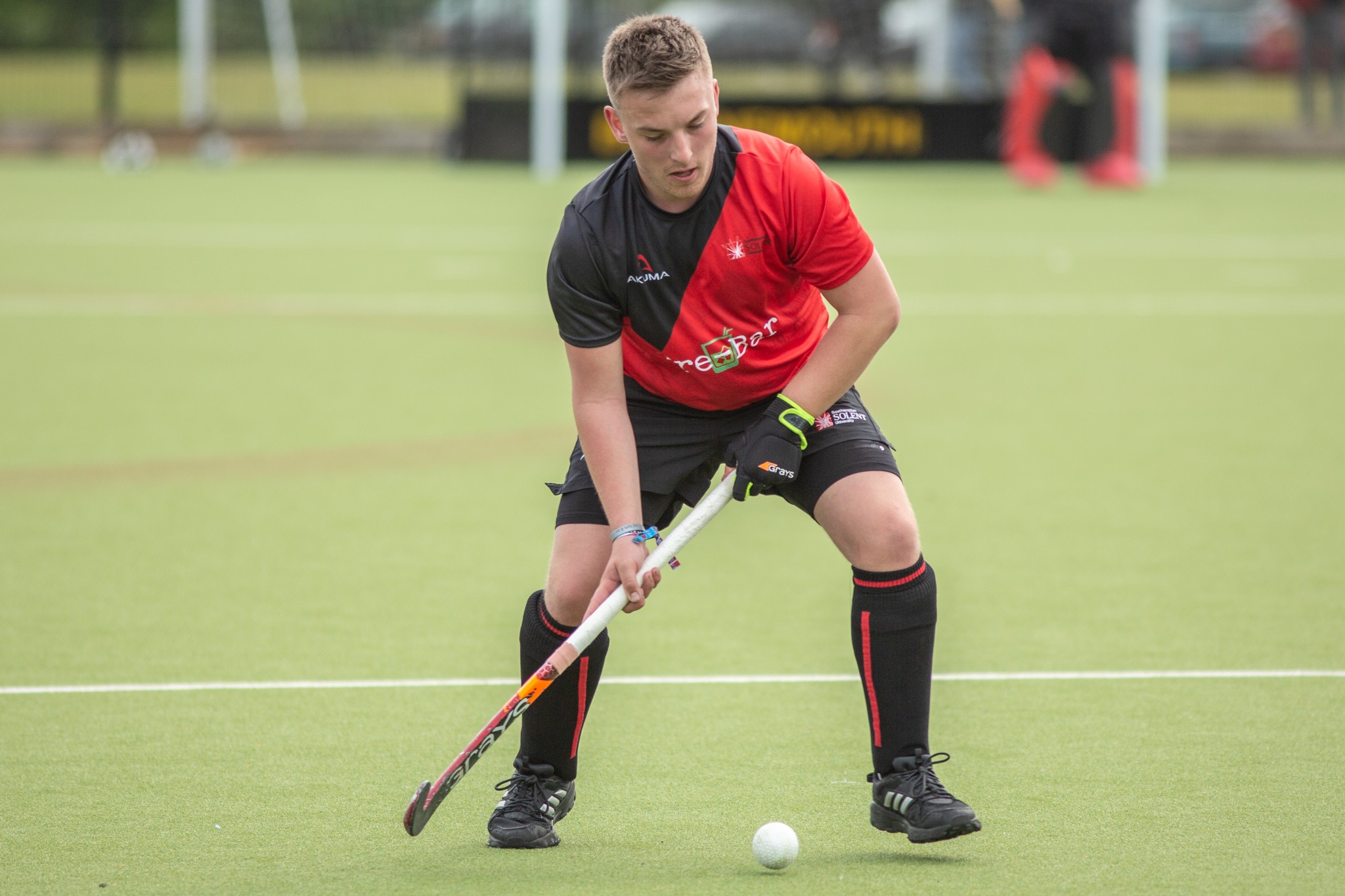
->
[481,16,983,846]
[877,0,1137,165]
[1284,0,1345,147]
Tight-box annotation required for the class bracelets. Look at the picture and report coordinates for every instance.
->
[609,524,681,571]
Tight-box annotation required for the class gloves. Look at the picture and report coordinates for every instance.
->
[725,392,817,502]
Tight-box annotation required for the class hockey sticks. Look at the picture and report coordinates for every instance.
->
[404,465,736,836]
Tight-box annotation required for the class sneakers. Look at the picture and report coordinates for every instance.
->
[870,746,981,844]
[485,769,576,849]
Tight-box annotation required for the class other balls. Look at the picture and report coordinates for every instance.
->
[752,822,799,871]
